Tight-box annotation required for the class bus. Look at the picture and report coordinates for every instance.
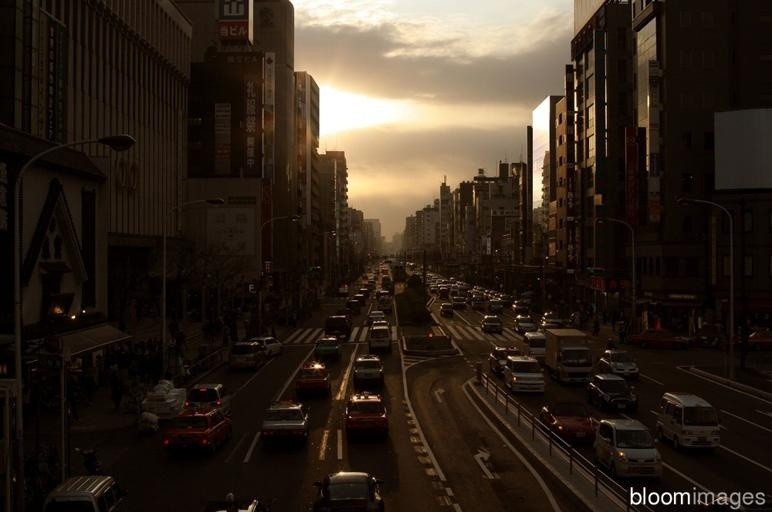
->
[625,288,772,351]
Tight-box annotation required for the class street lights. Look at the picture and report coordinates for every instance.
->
[259,214,300,336]
[15,134,136,510]
[566,216,635,317]
[676,198,737,378]
[162,197,225,379]
